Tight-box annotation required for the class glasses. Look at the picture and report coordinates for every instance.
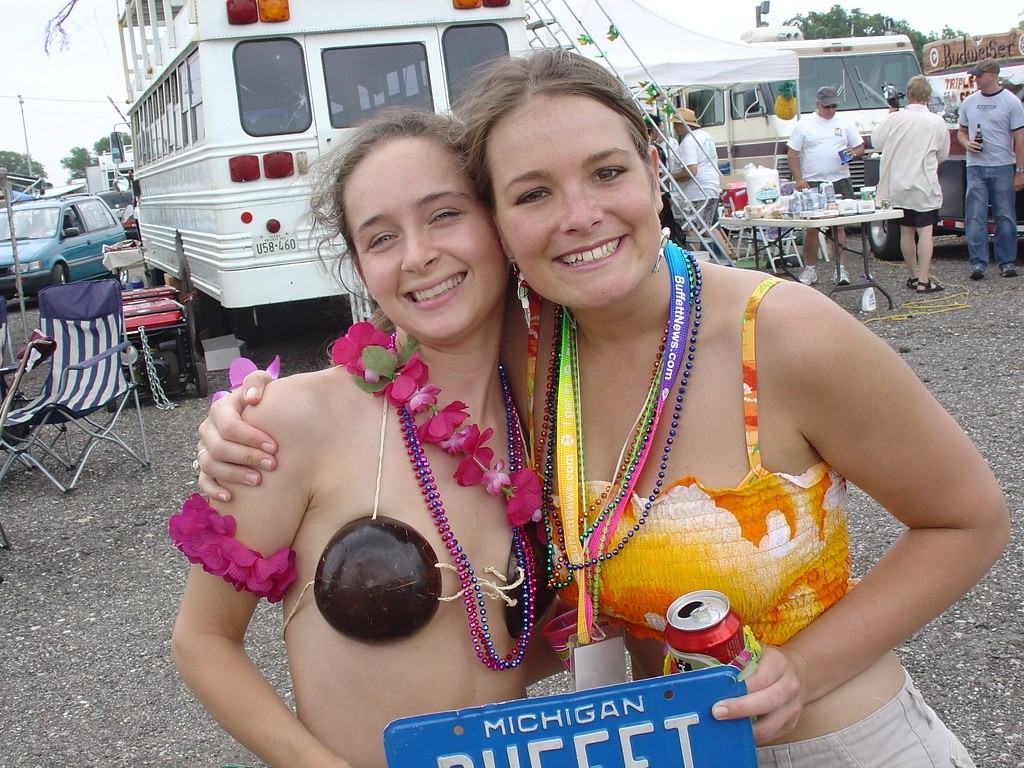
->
[820,104,838,108]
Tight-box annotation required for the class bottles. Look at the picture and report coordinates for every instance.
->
[716,203,725,217]
[974,124,983,152]
[862,192,868,200]
[944,91,959,107]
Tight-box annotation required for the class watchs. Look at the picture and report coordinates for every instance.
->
[1016,167,1024,173]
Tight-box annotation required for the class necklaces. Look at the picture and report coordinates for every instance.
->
[541,236,705,592]
[387,330,537,667]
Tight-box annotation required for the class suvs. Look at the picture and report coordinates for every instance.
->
[0,193,130,296]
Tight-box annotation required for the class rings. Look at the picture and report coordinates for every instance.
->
[193,449,208,469]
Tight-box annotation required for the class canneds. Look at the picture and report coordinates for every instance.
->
[665,589,745,668]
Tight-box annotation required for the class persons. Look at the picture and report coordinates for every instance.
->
[168,103,555,768]
[870,73,950,295]
[786,85,866,287]
[640,114,695,252]
[660,105,722,251]
[956,58,1024,278]
[193,56,1010,768]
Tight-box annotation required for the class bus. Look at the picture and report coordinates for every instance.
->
[116,0,532,314]
[608,35,929,230]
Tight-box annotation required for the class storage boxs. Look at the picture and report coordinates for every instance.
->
[200,328,241,372]
[164,273,183,302]
[721,174,749,217]
[687,232,699,250]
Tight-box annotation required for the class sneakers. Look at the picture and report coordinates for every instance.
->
[999,263,1017,276]
[969,264,985,279]
[832,265,850,285]
[798,267,817,286]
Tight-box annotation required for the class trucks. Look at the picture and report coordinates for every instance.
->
[858,29,1024,260]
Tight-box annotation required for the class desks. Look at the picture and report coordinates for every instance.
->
[719,208,903,311]
[102,250,146,288]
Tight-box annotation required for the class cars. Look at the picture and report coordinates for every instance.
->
[91,190,143,244]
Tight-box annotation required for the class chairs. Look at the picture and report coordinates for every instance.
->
[0,278,151,551]
[63,215,75,229]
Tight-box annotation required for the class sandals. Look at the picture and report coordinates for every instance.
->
[917,279,945,293]
[906,277,918,289]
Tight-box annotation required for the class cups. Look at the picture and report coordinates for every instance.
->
[542,608,604,680]
[838,149,852,164]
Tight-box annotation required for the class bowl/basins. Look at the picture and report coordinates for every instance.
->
[735,211,745,217]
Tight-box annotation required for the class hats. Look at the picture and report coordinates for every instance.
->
[667,108,701,128]
[816,86,840,106]
[967,58,1000,76]
